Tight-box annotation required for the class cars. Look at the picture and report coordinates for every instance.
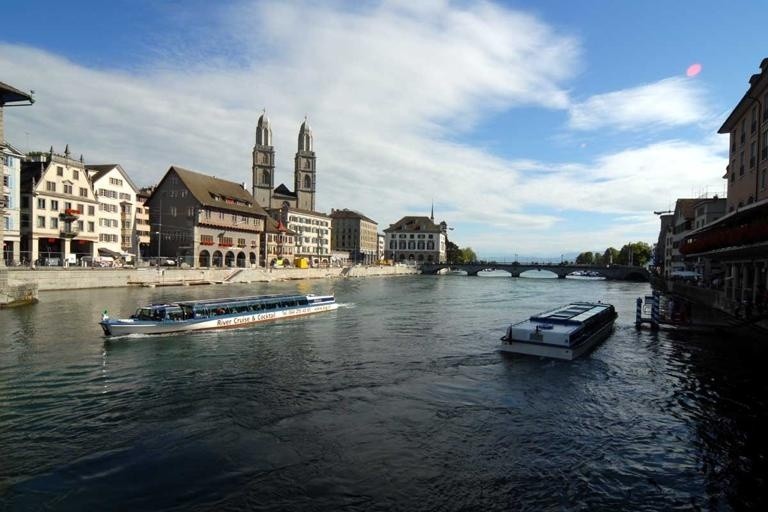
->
[160,257,175,266]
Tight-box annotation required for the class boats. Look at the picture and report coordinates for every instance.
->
[499,300,618,360]
[98,293,337,336]
